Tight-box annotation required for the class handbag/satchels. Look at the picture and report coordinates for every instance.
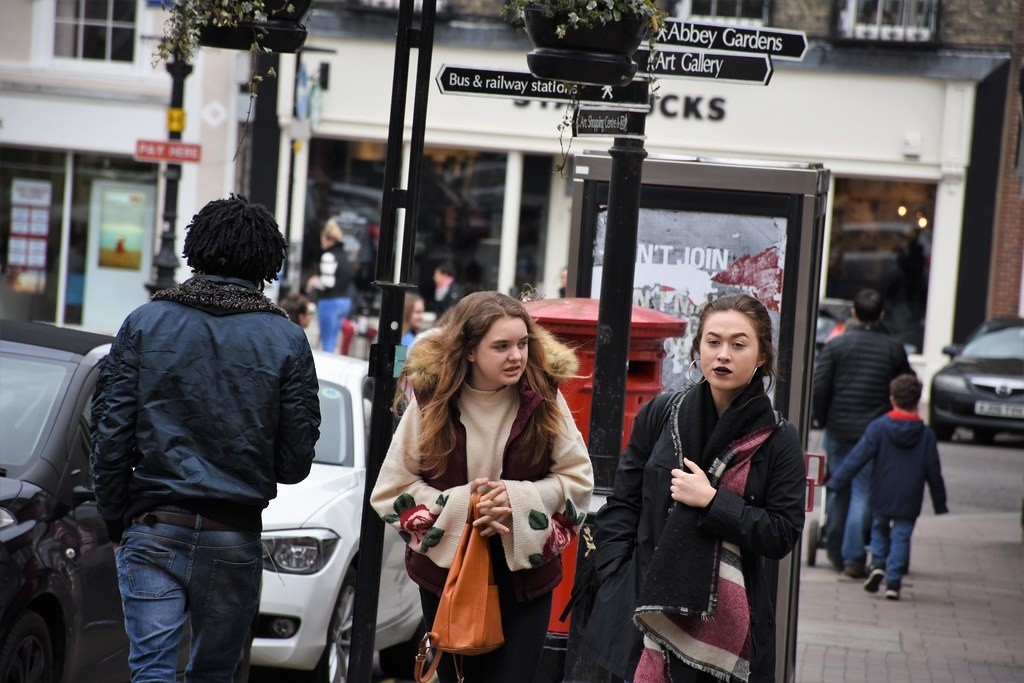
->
[414,494,505,683]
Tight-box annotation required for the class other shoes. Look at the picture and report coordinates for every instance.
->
[885,586,899,599]
[833,563,865,577]
[863,566,884,592]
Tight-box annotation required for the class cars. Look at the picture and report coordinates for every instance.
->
[597,223,931,382]
[248,349,432,683]
[1,318,192,683]
[929,315,1023,444]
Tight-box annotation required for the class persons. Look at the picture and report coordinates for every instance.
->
[372,292,594,683]
[401,290,426,349]
[560,293,806,683]
[431,261,462,314]
[811,289,917,582]
[280,295,310,330]
[308,220,352,353]
[825,374,948,600]
[89,199,321,683]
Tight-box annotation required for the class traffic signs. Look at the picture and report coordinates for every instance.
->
[572,104,647,141]
[644,16,801,61]
[634,46,773,86]
[435,62,652,106]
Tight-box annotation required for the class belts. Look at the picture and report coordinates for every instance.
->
[132,511,242,531]
